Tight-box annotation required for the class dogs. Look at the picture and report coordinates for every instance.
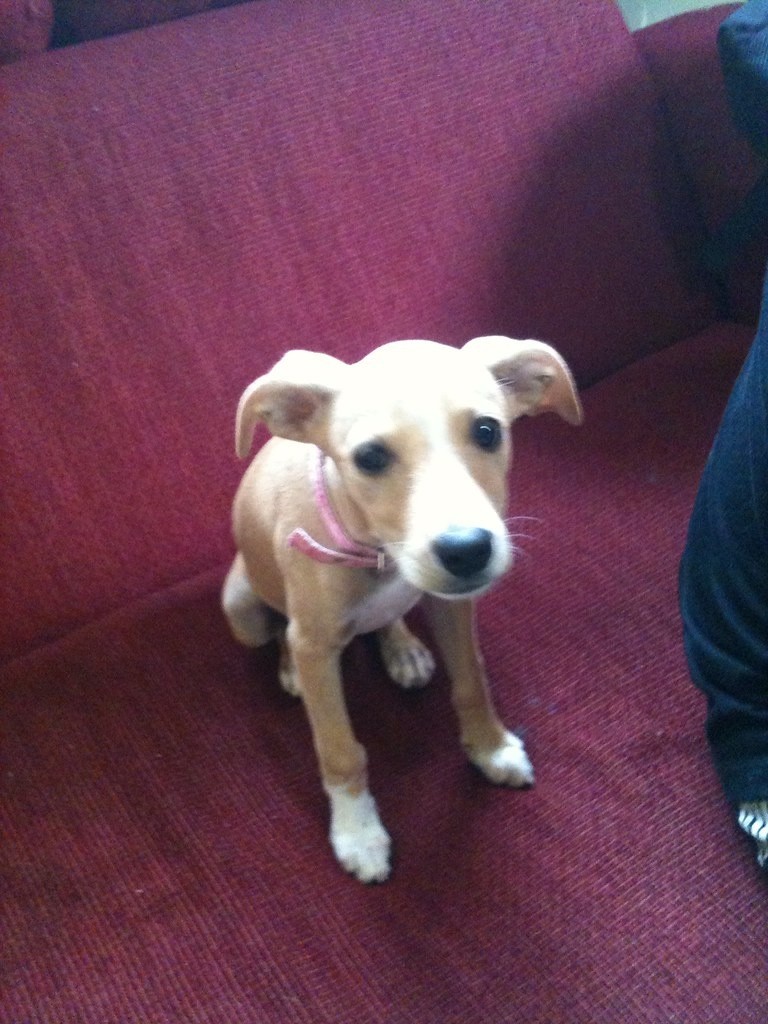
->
[221,335,585,883]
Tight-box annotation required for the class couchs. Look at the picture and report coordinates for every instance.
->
[0,0,768,1024]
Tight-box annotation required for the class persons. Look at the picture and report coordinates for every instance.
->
[677,0,768,865]
